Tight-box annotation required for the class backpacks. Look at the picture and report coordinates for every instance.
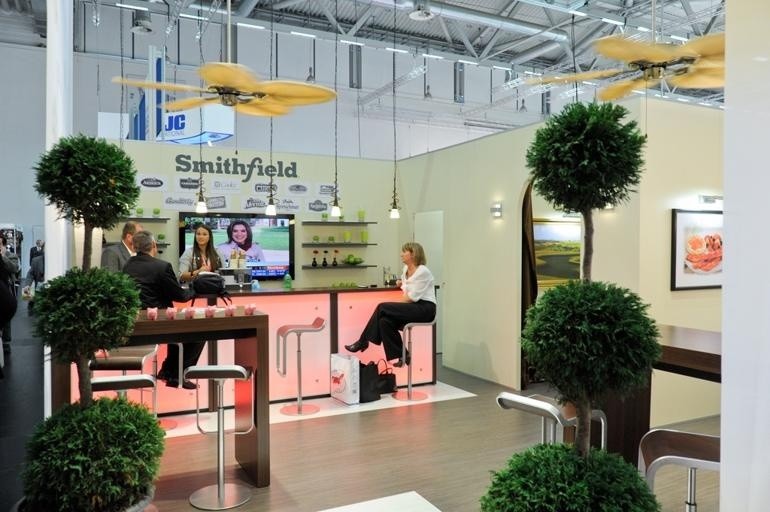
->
[189,269,226,298]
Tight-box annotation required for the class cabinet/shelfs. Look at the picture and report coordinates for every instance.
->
[301,221,378,268]
[97,217,171,247]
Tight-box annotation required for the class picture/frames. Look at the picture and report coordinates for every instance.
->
[532,218,585,289]
[670,208,726,296]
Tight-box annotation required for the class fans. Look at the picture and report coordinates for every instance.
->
[520,1,725,102]
[113,2,337,116]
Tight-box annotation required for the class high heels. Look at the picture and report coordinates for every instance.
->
[392,350,411,368]
[344,339,369,353]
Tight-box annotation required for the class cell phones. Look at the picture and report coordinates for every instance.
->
[359,285,368,288]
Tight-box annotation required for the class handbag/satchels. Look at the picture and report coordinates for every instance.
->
[359,361,380,403]
[329,353,361,405]
[376,371,397,394]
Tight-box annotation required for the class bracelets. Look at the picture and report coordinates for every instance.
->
[191,270,193,277]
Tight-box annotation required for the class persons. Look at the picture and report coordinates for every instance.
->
[0,230,23,351]
[179,224,222,281]
[344,243,437,367]
[23,242,45,314]
[216,220,265,263]
[101,221,143,272]
[29,239,44,265]
[123,230,200,388]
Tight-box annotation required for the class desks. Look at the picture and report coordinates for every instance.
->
[606,321,721,470]
[49,306,271,487]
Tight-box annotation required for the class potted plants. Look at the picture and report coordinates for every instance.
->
[9,127,156,511]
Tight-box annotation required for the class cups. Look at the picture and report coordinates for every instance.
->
[229,259,238,269]
[238,259,246,269]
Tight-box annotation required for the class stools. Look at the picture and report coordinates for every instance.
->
[277,316,326,416]
[89,374,156,420]
[392,302,439,400]
[89,343,158,405]
[146,340,187,432]
[497,392,608,453]
[640,412,720,511]
[185,365,255,512]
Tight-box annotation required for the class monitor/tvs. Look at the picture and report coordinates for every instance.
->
[179,212,295,282]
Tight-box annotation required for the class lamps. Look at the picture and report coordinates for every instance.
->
[195,0,207,215]
[330,1,342,217]
[389,3,401,222]
[264,2,276,218]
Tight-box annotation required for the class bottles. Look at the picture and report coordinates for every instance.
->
[283,271,292,289]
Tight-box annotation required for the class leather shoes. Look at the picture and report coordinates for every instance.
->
[166,379,199,390]
[156,368,166,383]
